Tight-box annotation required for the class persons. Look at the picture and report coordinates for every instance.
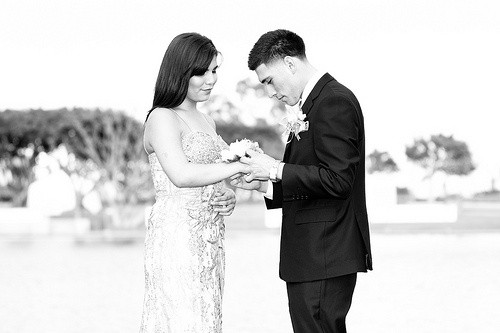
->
[139,32,252,332]
[231,29,372,333]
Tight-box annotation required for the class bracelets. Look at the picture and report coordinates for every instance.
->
[268,159,282,182]
[223,186,239,203]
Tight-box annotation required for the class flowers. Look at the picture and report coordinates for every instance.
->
[220,137,263,164]
[277,103,310,144]
